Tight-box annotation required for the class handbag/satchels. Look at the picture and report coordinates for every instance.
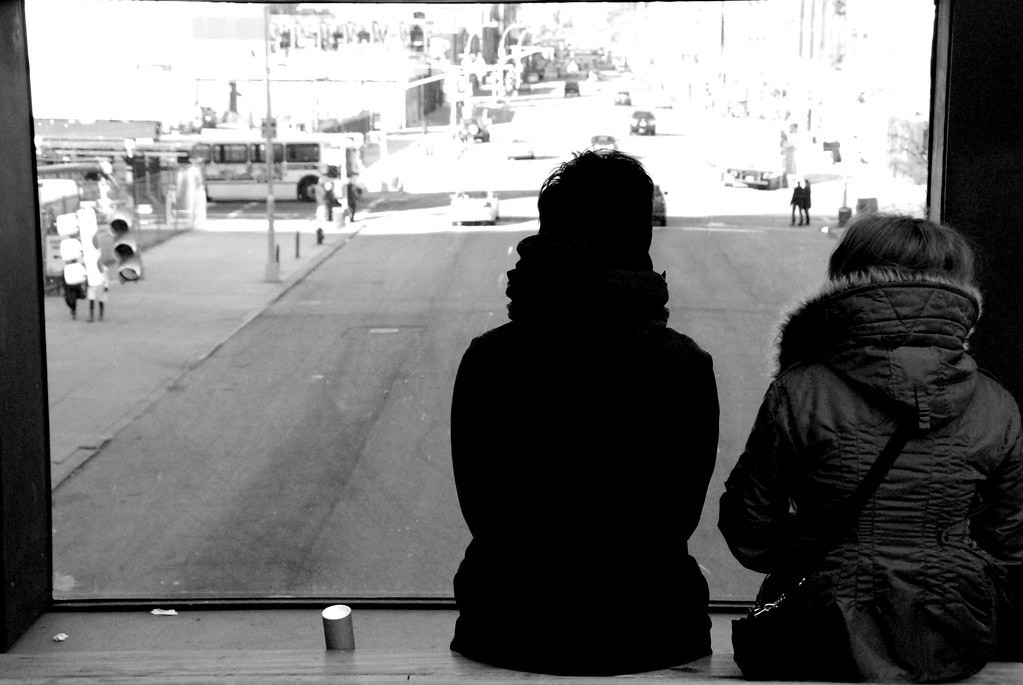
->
[731,571,864,682]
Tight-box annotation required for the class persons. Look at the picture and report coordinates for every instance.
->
[325,188,341,220]
[717,217,1023,685]
[347,184,361,222]
[789,179,812,225]
[451,148,721,677]
[60,258,108,323]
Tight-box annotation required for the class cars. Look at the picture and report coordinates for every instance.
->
[563,81,580,98]
[651,185,669,226]
[589,134,620,157]
[506,135,535,162]
[614,90,632,106]
[630,110,656,136]
[449,186,500,225]
[459,117,491,145]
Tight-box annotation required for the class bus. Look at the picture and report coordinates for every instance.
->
[39,126,365,287]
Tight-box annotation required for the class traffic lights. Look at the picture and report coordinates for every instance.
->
[106,205,145,286]
[57,207,104,290]
